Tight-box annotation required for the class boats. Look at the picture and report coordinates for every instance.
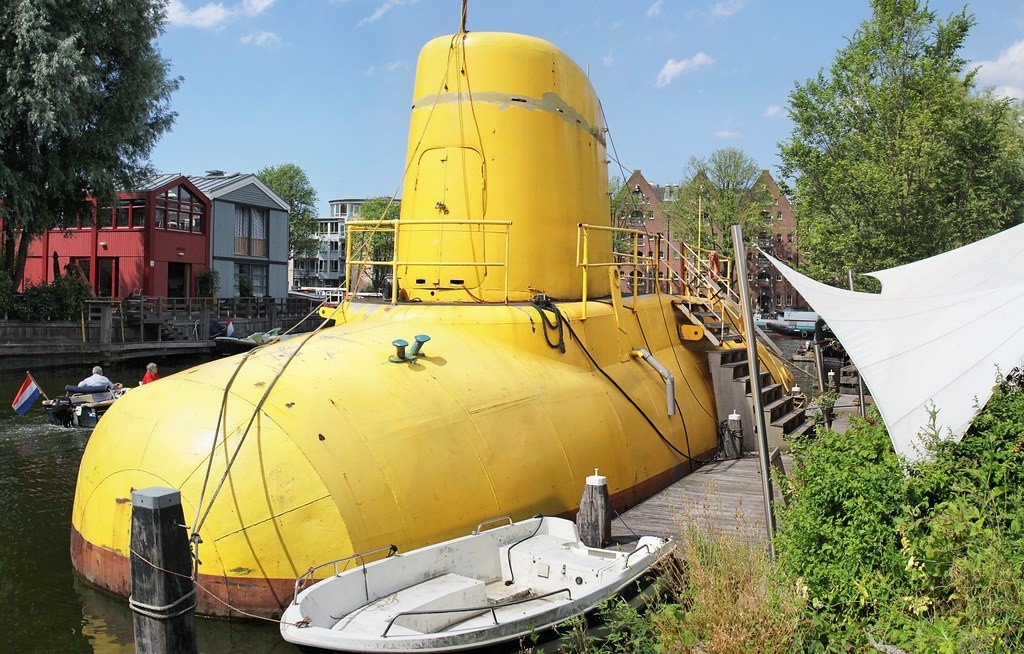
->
[280,515,678,654]
[213,327,310,357]
[39,385,136,428]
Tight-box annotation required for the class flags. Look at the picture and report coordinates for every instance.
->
[11,374,42,418]
[227,316,235,337]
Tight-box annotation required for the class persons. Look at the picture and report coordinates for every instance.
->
[78,366,123,403]
[142,362,159,385]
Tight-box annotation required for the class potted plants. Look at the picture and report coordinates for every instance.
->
[233,273,257,318]
[197,267,221,312]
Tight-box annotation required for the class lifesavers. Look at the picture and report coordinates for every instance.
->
[708,251,720,282]
